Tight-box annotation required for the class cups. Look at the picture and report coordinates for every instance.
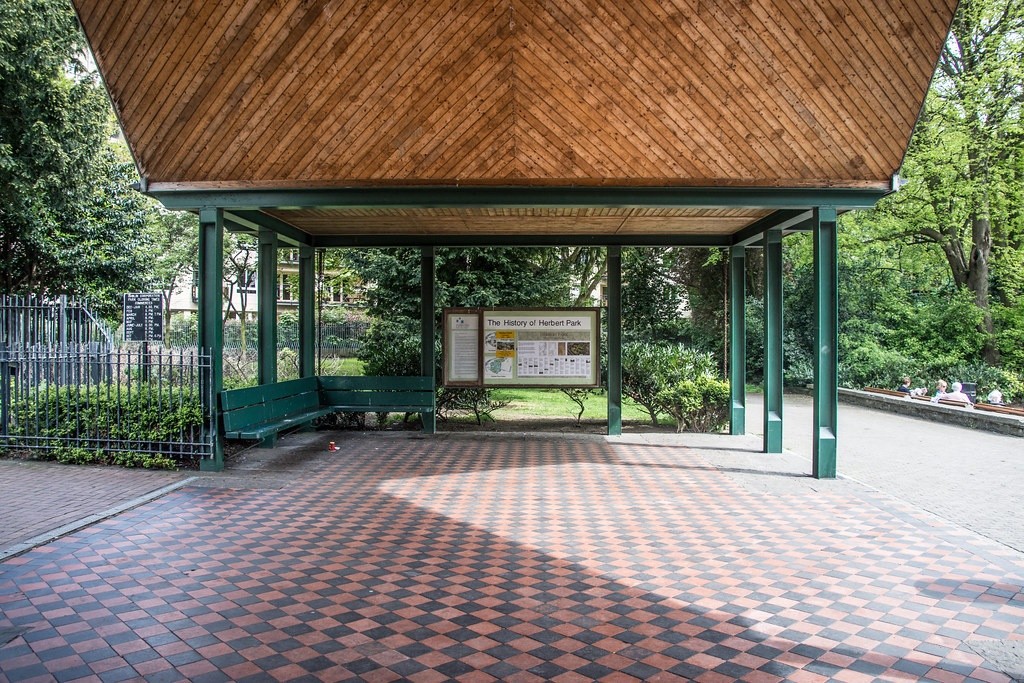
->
[328,441,336,452]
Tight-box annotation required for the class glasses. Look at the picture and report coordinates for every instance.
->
[941,385,947,388]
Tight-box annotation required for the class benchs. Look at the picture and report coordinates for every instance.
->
[221,375,434,462]
[864,387,909,398]
[912,395,969,408]
[973,403,1024,416]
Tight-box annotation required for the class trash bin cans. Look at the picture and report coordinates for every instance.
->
[960,383,977,404]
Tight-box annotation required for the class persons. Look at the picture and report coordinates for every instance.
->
[897,377,911,395]
[937,382,973,408]
[931,379,948,397]
